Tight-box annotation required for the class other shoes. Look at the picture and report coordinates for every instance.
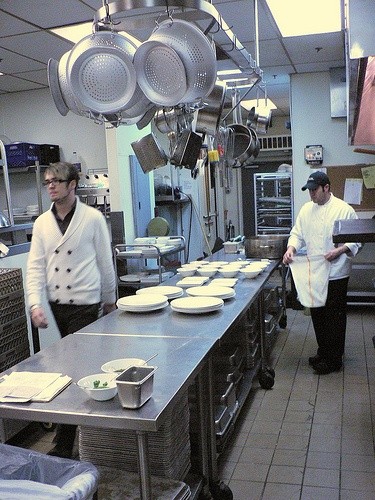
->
[309,354,342,372]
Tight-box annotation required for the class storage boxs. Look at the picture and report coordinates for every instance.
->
[0,142,60,169]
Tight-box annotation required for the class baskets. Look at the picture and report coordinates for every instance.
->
[169,129,202,170]
[130,132,168,174]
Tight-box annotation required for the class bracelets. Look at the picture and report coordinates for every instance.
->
[30,305,39,311]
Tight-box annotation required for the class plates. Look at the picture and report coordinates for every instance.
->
[119,265,174,282]
[134,236,181,249]
[116,277,236,315]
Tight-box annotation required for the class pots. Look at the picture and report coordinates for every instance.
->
[147,206,170,236]
[226,83,271,168]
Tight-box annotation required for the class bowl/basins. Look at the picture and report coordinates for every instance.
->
[101,359,147,375]
[76,373,118,401]
[2,207,24,218]
[176,261,270,278]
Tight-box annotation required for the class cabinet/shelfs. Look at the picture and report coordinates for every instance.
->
[112,236,186,300]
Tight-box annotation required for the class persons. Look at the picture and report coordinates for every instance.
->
[283,172,363,375]
[26,162,116,458]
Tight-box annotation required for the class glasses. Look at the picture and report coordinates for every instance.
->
[42,179,69,186]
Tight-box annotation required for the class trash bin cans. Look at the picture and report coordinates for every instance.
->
[0,443,100,500]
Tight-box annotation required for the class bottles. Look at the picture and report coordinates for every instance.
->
[69,152,82,187]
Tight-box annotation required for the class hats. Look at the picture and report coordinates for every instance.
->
[301,170,331,192]
